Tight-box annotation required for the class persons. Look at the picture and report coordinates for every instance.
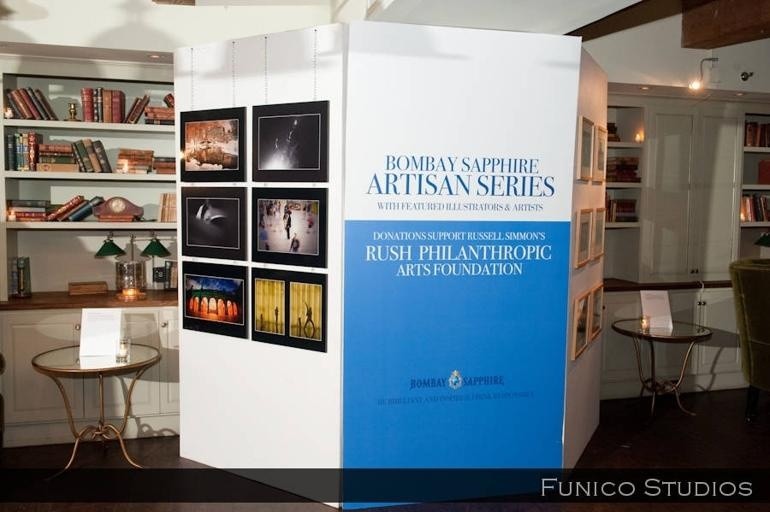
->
[258,200,315,252]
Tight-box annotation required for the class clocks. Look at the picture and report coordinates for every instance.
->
[90,195,144,222]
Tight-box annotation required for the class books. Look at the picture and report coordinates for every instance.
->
[606,156,642,182]
[6,133,176,175]
[605,193,639,222]
[6,194,105,221]
[158,193,178,222]
[741,193,770,222]
[6,87,56,121]
[81,87,175,124]
[12,256,31,297]
[744,120,770,147]
[607,123,620,141]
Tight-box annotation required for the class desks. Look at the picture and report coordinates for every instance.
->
[608,316,714,451]
[30,344,165,485]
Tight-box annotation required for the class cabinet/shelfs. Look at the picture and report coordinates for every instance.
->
[737,98,770,263]
[598,91,655,290]
[153,288,180,443]
[1,42,181,312]
[3,289,87,455]
[81,282,155,446]
[644,95,749,285]
[598,287,752,401]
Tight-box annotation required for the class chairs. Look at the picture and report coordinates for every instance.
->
[727,256,770,432]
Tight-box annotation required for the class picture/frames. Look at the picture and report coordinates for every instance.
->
[570,111,611,366]
[177,98,333,356]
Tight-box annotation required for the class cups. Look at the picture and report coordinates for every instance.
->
[115,339,130,363]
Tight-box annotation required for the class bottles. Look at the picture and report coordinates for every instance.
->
[641,315,651,331]
